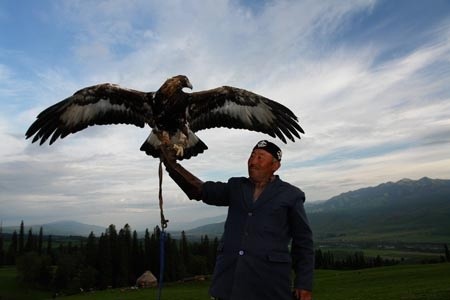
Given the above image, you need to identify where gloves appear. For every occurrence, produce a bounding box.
[158,144,203,200]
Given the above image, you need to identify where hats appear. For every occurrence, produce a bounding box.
[253,140,282,161]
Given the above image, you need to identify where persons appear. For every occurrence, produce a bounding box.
[158,140,316,300]
